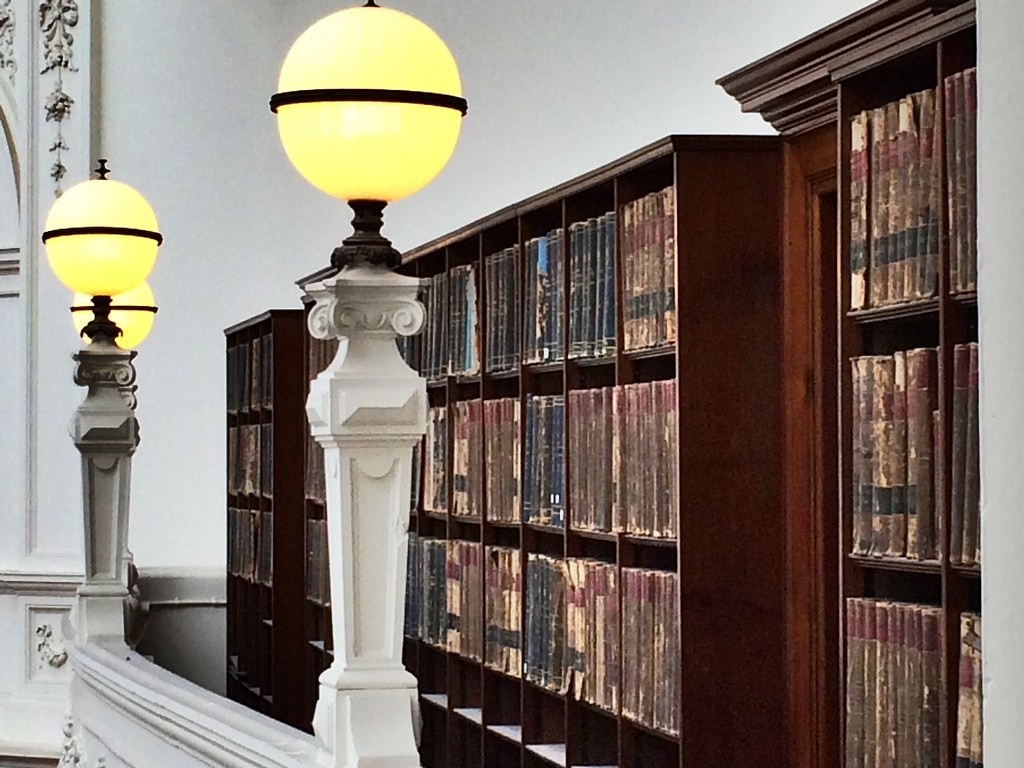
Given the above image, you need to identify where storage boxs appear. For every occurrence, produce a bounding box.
[223,0,982,768]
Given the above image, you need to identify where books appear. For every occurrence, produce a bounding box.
[305,336,339,606]
[403,186,680,740]
[227,335,273,588]
[847,65,983,768]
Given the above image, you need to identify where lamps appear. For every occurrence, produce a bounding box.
[270,1,469,268]
[41,159,163,351]
